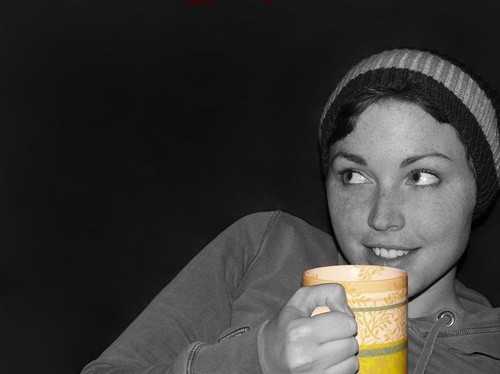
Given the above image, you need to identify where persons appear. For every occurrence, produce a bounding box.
[80,47,500,373]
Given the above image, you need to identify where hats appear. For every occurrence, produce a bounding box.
[317,47,499,220]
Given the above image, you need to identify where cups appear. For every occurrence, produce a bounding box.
[302,265,408,374]
